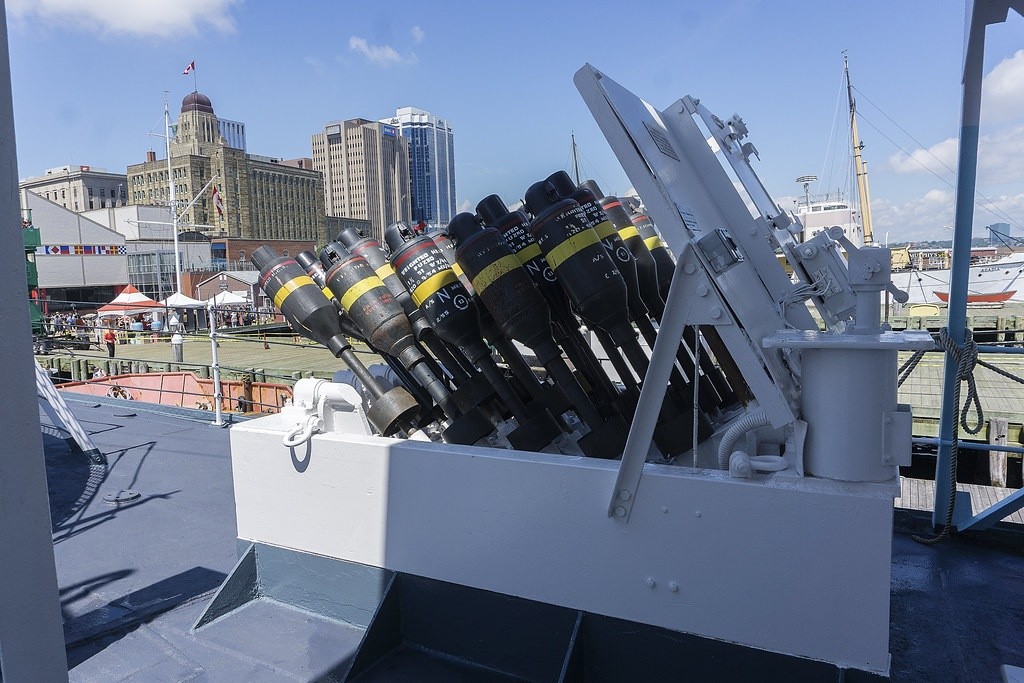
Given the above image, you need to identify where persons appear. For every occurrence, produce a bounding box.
[183,312,189,330]
[216,309,256,326]
[104,327,116,359]
[132,316,148,331]
[45,311,95,333]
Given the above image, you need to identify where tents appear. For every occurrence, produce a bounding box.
[204,289,254,318]
[97,283,165,334]
[156,291,208,329]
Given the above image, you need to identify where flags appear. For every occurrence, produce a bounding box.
[213,186,224,217]
